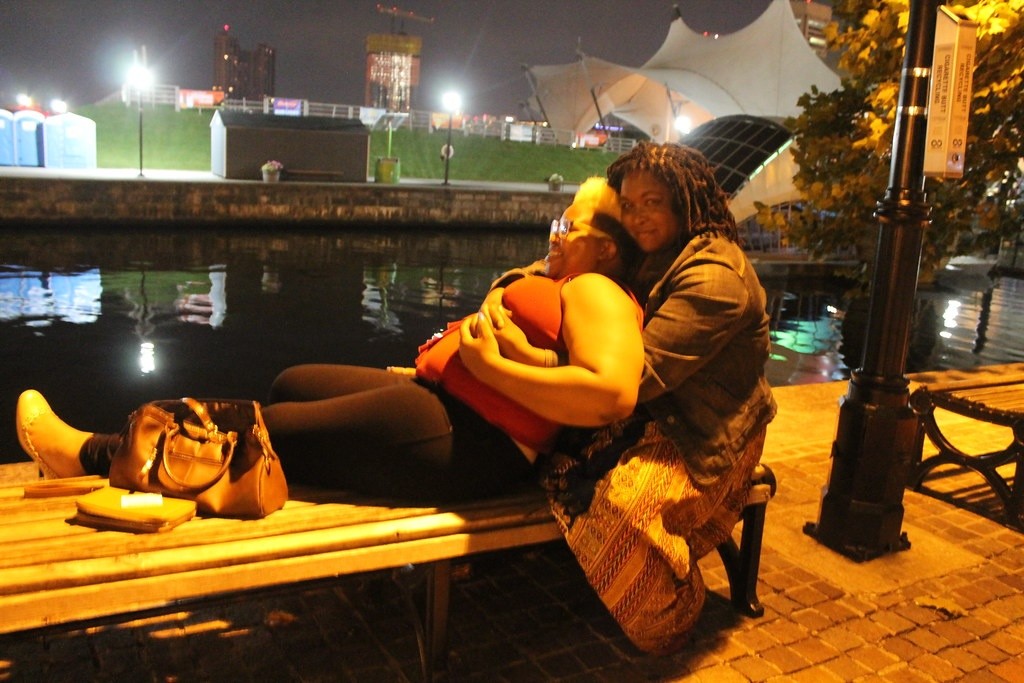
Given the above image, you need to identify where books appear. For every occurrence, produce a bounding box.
[24,475,196,535]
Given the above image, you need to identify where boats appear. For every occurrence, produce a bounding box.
[936,251,1000,291]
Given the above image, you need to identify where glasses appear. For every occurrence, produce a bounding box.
[550,219,600,240]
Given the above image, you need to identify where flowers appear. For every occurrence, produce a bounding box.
[262,160,283,174]
[549,173,563,183]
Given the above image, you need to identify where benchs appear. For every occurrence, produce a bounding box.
[912,375,1024,513]
[1,459,778,683]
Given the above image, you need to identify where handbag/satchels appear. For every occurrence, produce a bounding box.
[108,396,288,518]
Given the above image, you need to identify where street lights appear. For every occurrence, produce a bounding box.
[133,68,152,179]
[441,90,461,186]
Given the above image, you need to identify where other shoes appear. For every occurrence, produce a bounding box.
[17,390,66,481]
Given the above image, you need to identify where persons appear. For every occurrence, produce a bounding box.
[976,196,1019,232]
[459,143,777,653]
[15,176,644,507]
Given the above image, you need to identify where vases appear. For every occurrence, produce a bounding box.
[263,171,280,182]
[548,181,560,191]
